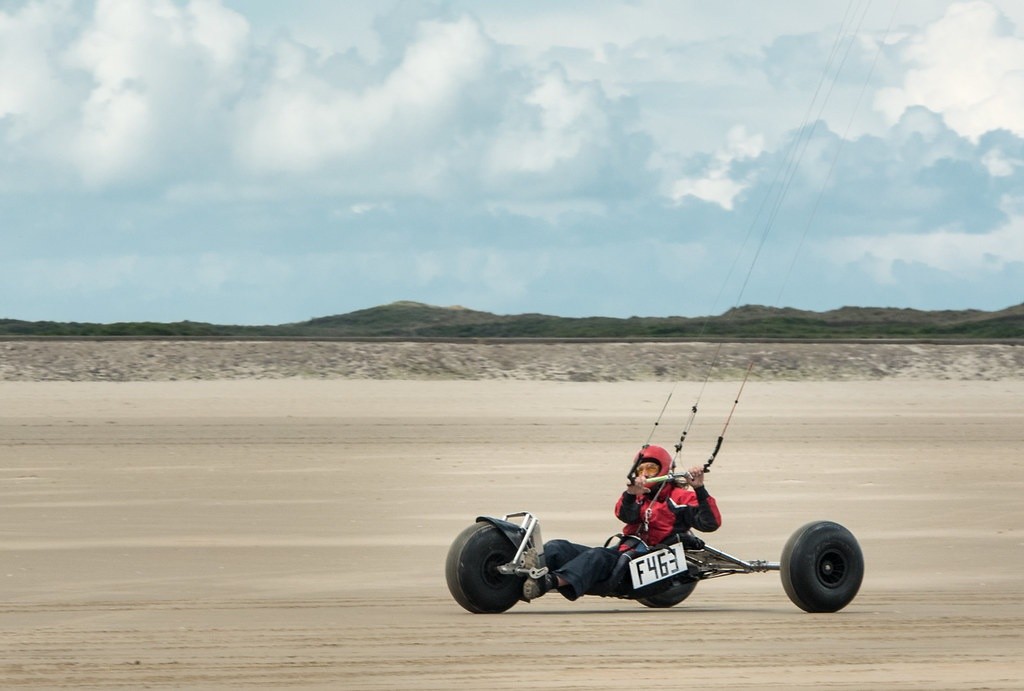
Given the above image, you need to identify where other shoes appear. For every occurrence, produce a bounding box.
[523,548,545,599]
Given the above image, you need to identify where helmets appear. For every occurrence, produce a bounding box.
[634,446,673,488]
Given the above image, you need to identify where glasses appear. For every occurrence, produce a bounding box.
[637,464,658,475]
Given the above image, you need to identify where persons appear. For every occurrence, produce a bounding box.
[522,445,721,601]
